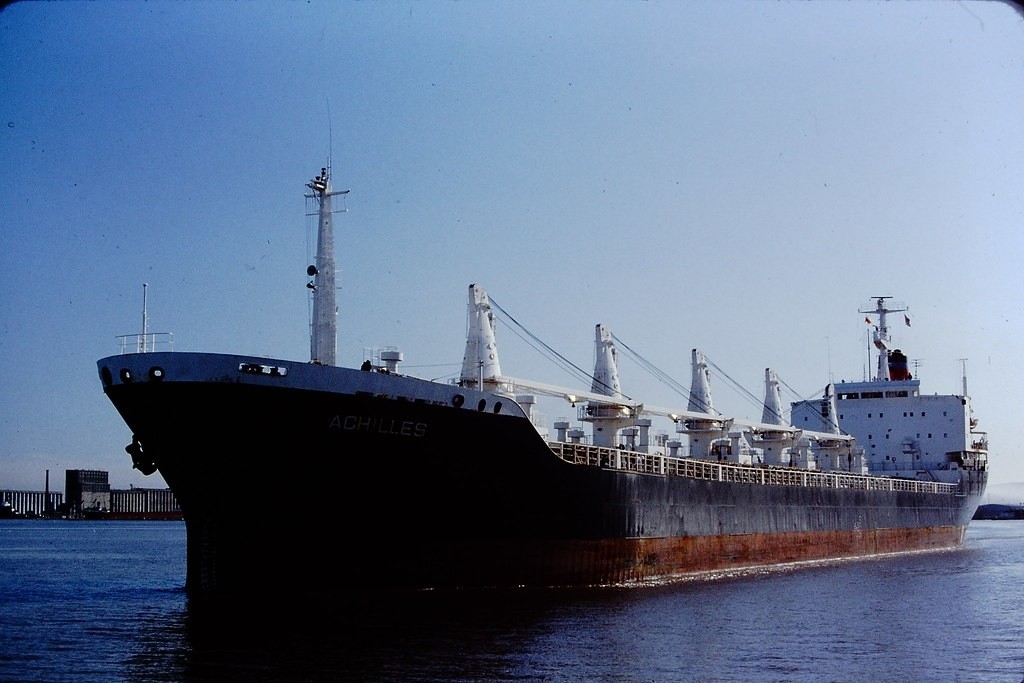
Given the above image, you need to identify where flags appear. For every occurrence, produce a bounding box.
[865,318,871,324]
[905,315,911,327]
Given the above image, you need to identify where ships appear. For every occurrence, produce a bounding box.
[96,99,987,609]
